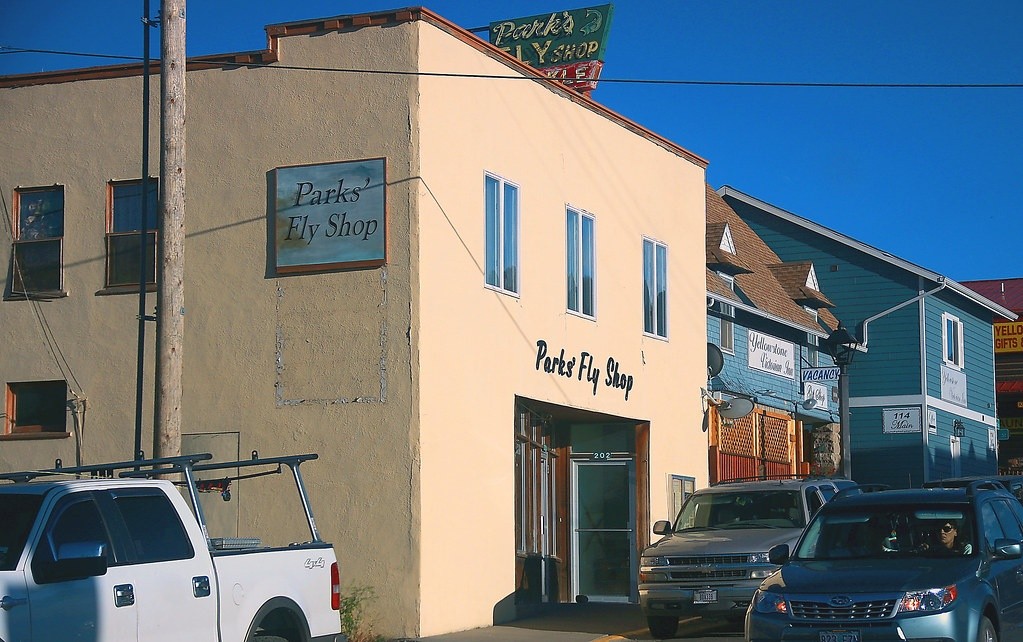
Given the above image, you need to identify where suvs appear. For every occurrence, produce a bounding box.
[639,474,865,639]
[744,473,1023,642]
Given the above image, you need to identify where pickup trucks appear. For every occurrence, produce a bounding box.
[0,450,344,642]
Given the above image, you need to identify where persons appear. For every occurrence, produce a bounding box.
[922,519,971,554]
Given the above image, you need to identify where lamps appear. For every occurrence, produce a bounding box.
[700,387,755,419]
[798,319,859,395]
[954,419,965,437]
[793,398,817,410]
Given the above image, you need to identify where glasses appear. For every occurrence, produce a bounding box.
[937,525,955,532]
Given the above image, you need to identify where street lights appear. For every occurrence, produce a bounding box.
[824,319,859,482]
